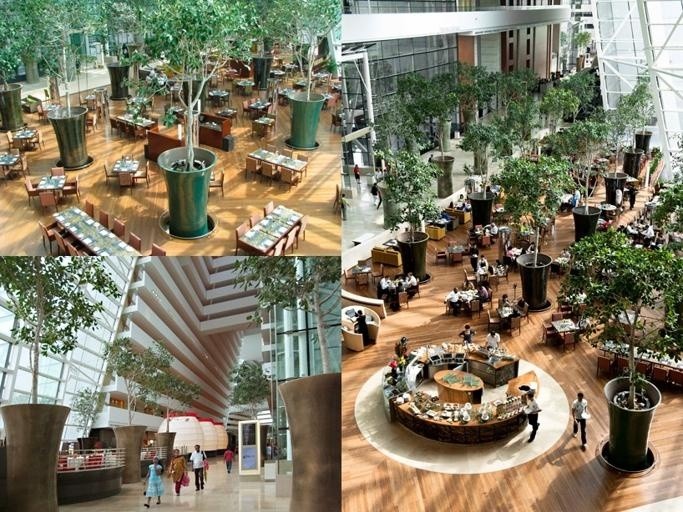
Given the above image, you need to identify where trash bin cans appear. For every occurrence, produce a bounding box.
[223,135,234,151]
[263,460,278,480]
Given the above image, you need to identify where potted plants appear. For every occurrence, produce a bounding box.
[100,339,201,484]
[364,53,657,311]
[556,227,683,479]
[1,258,124,510]
[1,1,343,240]
[228,358,273,466]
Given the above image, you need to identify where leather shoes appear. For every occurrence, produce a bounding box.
[196,487,204,491]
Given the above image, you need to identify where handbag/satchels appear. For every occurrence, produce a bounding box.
[180,474,190,486]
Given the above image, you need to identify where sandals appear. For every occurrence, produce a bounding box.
[144,502,160,508]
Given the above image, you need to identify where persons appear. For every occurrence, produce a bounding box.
[355,310,370,346]
[543,184,582,214]
[375,271,419,311]
[430,183,538,330]
[570,392,590,451]
[639,225,655,243]
[457,324,476,343]
[650,242,660,251]
[341,193,351,221]
[655,328,678,357]
[222,446,235,472]
[523,390,542,442]
[189,444,208,490]
[144,67,182,97]
[265,441,287,461]
[483,330,501,349]
[625,222,639,241]
[560,248,571,265]
[370,183,379,206]
[574,314,592,343]
[352,164,360,184]
[142,455,164,508]
[166,448,188,496]
[627,185,637,211]
[428,154,437,178]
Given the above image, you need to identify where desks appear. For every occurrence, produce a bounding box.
[380,343,538,443]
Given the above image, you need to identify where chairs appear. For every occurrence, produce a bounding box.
[237,204,305,256]
[54,229,90,257]
[595,322,683,384]
[541,311,585,352]
